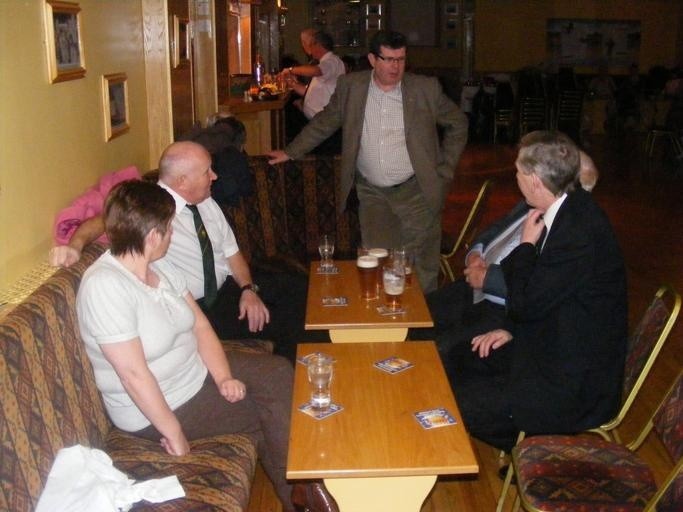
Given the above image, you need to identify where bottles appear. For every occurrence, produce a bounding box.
[253,54,264,86]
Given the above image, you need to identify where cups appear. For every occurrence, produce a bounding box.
[306,353,334,412]
[356,244,415,314]
[317,233,334,272]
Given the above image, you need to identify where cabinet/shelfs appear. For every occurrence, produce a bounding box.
[188,0,297,157]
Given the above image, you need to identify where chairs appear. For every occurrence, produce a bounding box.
[458,66,683,158]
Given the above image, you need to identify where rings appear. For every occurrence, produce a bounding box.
[240,390,244,393]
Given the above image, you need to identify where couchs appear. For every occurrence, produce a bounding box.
[0,116,318,512]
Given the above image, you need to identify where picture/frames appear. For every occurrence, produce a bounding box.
[43,0,87,84]
[101,72,132,144]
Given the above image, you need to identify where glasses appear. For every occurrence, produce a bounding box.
[376,55,406,64]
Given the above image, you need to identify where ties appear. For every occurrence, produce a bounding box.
[536,215,546,255]
[472,218,528,304]
[185,204,218,311]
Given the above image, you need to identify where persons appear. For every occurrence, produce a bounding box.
[263,27,471,296]
[75,175,340,512]
[46,137,331,366]
[276,31,346,153]
[592,59,683,132]
[408,148,599,357]
[445,126,629,485]
[288,28,321,119]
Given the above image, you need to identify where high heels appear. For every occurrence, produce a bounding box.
[290,477,338,511]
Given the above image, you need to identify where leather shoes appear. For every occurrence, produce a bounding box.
[497,463,517,485]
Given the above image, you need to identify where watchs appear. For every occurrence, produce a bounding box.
[289,66,293,73]
[241,282,261,294]
